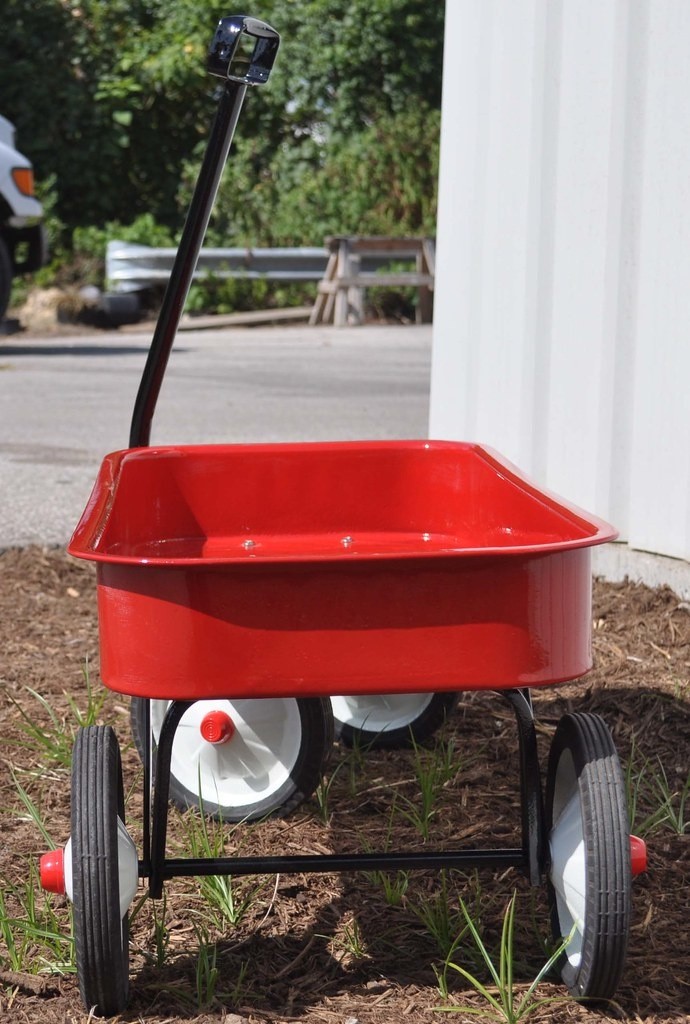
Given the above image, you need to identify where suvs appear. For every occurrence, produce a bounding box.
[0,114,46,319]
